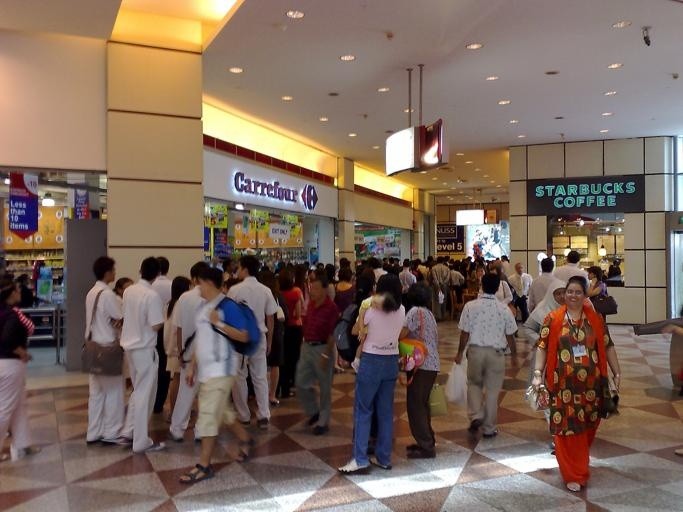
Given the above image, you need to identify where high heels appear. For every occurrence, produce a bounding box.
[270,400,281,408]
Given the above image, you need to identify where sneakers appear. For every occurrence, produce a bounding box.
[147,441,165,451]
[468,420,480,432]
[407,444,420,450]
[407,450,435,458]
[352,357,360,375]
[257,418,268,428]
[313,425,329,435]
[369,454,392,469]
[482,432,496,438]
[309,414,318,424]
[338,459,369,475]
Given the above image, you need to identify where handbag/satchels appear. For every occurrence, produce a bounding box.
[603,376,619,419]
[81,341,124,374]
[429,383,447,416]
[509,303,516,316]
[591,296,617,314]
[277,305,285,322]
[525,384,551,410]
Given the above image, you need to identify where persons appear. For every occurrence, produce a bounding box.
[660,307,683,456]
[0,272,38,460]
[86,247,622,492]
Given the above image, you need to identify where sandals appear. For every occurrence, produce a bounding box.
[566,482,580,491]
[180,464,214,483]
[236,439,255,462]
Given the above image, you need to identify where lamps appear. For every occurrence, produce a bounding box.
[42,192,55,207]
[564,236,571,256]
[598,229,606,256]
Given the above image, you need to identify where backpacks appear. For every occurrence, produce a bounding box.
[211,297,260,355]
[399,307,427,385]
[334,303,361,366]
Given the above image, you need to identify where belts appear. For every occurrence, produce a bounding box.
[308,341,326,345]
